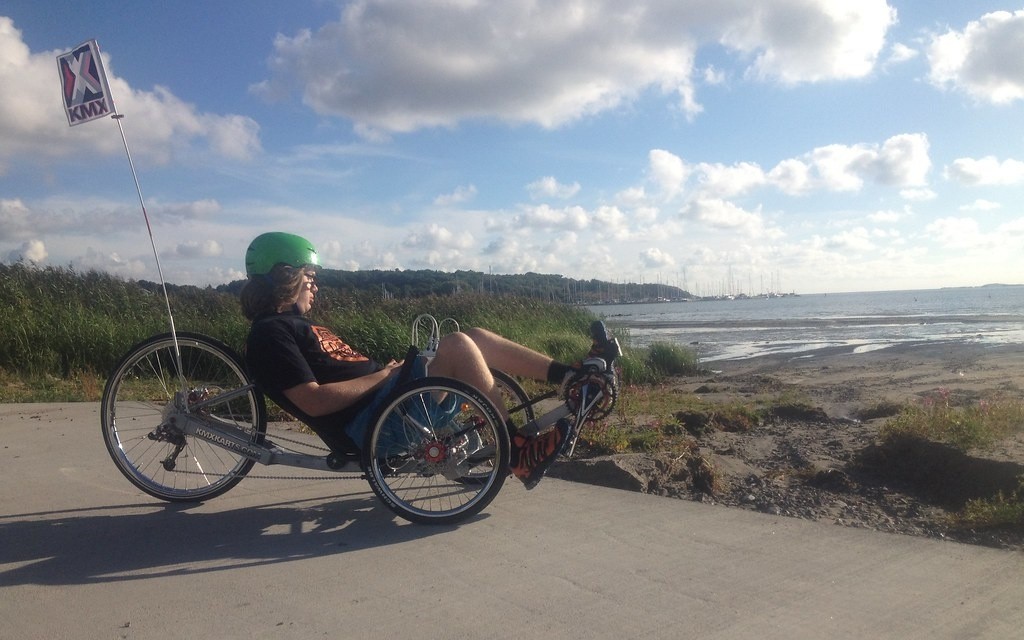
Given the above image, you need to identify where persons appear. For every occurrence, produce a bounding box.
[240,232,606,490]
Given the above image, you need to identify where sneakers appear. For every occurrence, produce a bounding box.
[512,417,571,490]
[574,320,609,402]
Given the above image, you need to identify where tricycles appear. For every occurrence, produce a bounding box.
[100,313,622,527]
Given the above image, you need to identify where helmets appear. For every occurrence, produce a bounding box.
[245,231,322,278]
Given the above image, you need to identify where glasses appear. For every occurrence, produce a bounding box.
[301,271,317,286]
